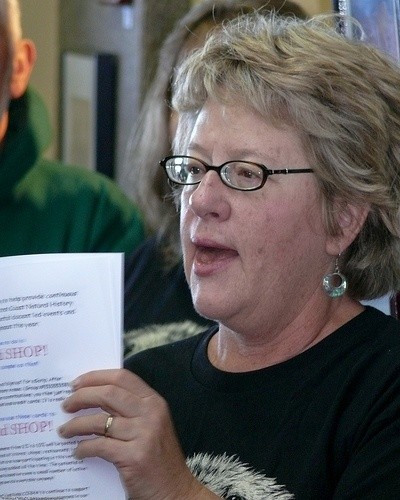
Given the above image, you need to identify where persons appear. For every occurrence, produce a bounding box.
[0,1,162,329]
[55,10,400,500]
[123,0,313,357]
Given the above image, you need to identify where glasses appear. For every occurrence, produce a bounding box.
[158,155,318,192]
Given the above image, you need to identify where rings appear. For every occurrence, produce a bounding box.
[104,416,114,435]
